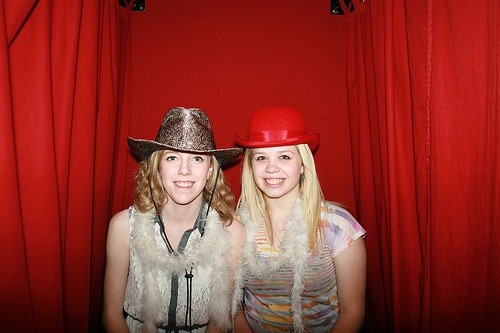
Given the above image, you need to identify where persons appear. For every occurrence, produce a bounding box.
[231,106,367,333]
[103,106,245,333]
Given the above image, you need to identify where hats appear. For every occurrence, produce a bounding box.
[234,104,321,154]
[127,105,244,166]
